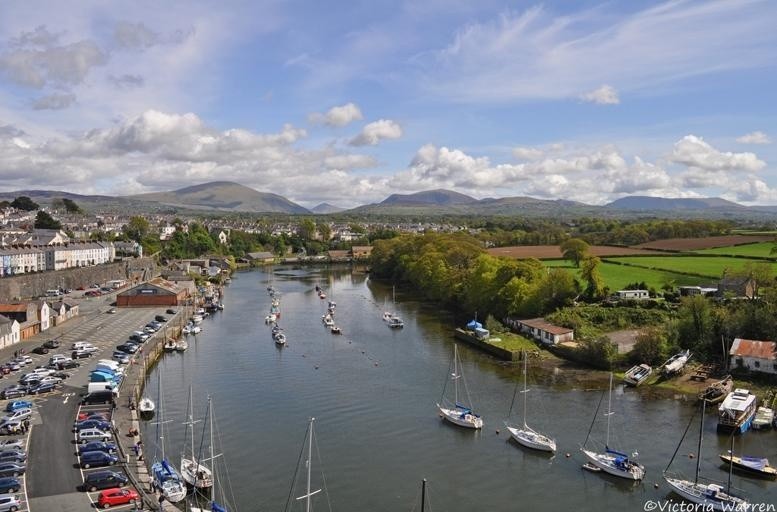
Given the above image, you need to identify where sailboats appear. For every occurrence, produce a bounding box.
[501,348,558,455]
[663,395,753,511]
[408,477,439,510]
[434,344,484,431]
[578,372,646,482]
[138,355,340,511]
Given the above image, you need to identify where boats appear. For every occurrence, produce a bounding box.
[719,447,776,478]
[163,277,231,354]
[690,361,719,380]
[697,373,776,437]
[382,310,403,329]
[264,284,287,347]
[315,285,340,334]
[623,364,652,386]
[656,347,694,378]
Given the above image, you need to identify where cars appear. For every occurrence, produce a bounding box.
[109,301,116,306]
[44,279,125,298]
[107,307,116,314]
[129,315,167,342]
[0,339,141,511]
[166,308,177,314]
